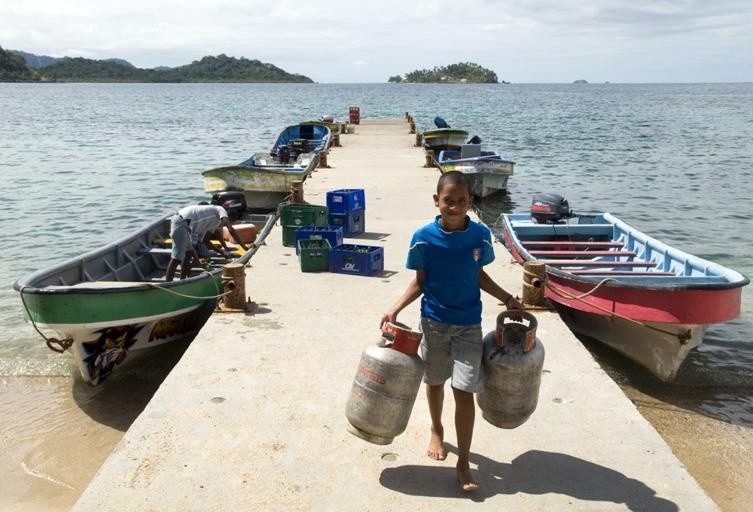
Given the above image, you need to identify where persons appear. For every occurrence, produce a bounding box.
[189,202,227,258]
[379,172,523,492]
[165,200,257,282]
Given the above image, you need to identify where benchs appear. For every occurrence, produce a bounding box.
[139,238,250,262]
[517,238,677,275]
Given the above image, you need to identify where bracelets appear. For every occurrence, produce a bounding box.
[504,295,512,305]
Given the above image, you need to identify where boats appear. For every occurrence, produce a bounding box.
[11,202,277,388]
[421,126,517,199]
[200,117,344,211]
[500,210,751,386]
